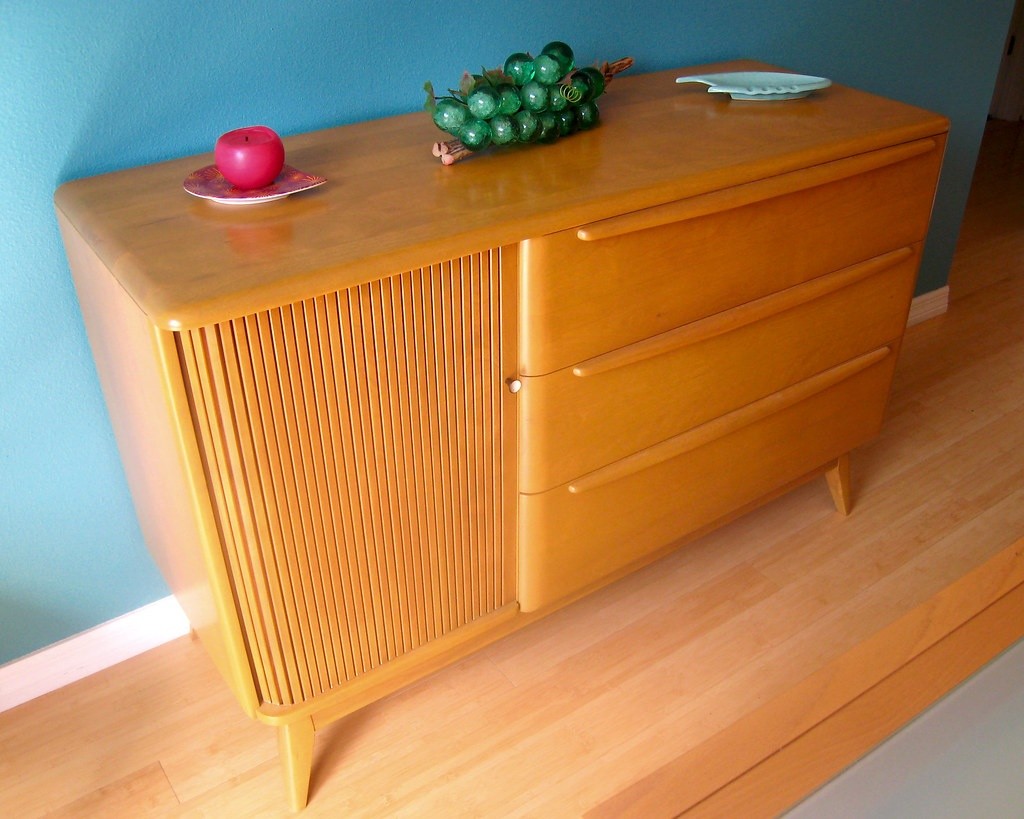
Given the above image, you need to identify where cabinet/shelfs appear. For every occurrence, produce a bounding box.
[51,61,948,816]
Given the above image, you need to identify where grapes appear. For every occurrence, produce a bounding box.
[432,41,606,150]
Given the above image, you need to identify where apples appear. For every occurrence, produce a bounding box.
[215,125,285,189]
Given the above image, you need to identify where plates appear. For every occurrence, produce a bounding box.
[676,71,831,101]
[183,162,327,204]
